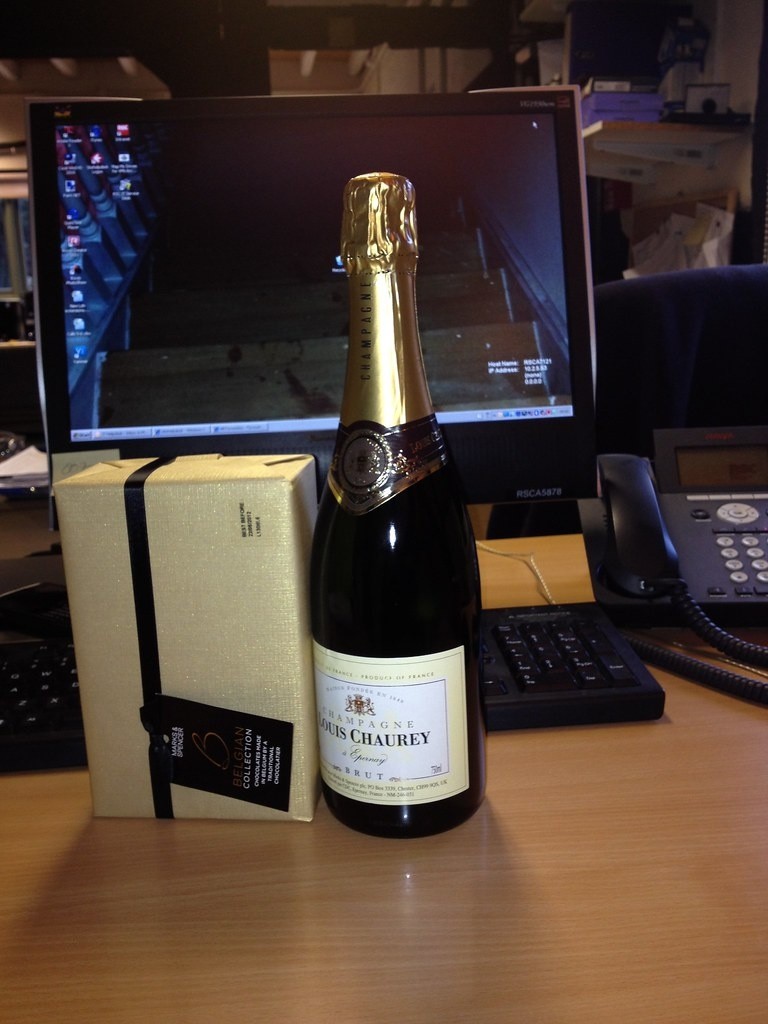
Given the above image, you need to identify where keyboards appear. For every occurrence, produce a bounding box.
[1,600,667,775]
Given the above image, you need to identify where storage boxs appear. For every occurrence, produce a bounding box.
[53,453,320,823]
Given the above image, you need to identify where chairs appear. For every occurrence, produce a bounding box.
[487,264,768,539]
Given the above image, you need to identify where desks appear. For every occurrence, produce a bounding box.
[0,502,768,1024]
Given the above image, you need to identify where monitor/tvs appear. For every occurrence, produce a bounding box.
[23,84,597,533]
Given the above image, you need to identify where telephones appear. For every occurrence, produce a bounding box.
[576,426,768,627]
